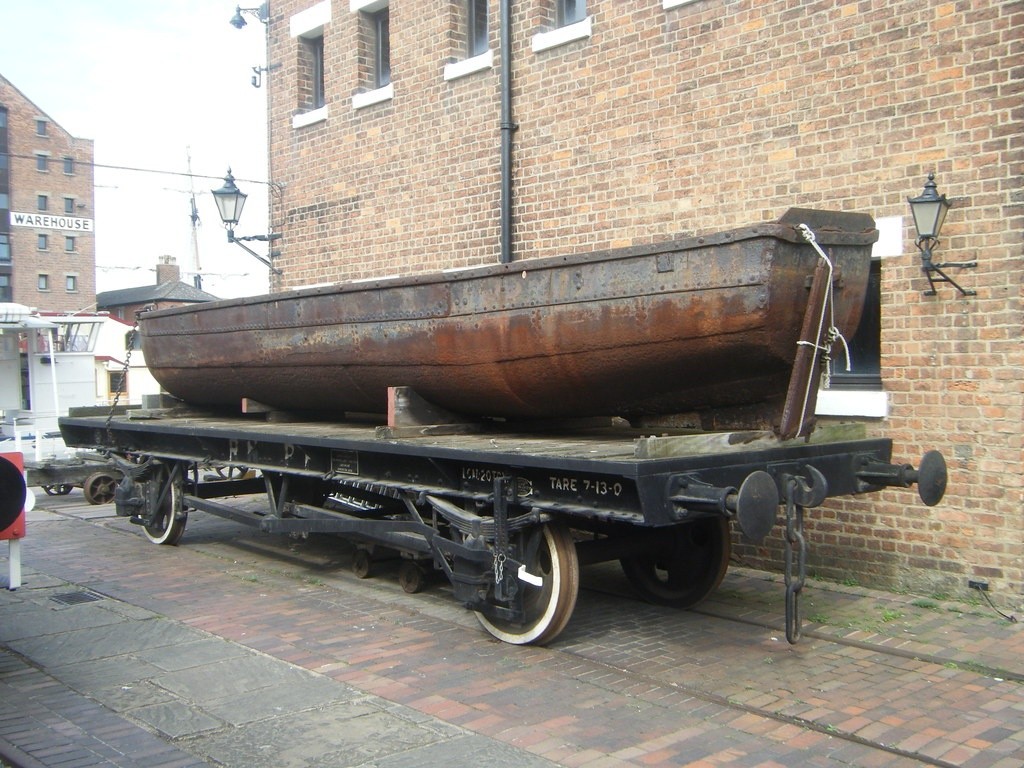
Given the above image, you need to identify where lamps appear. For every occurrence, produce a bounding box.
[211,168,283,274]
[907,172,977,296]
[252,63,281,88]
[229,3,267,29]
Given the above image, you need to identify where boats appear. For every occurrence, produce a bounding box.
[137,205,880,433]
